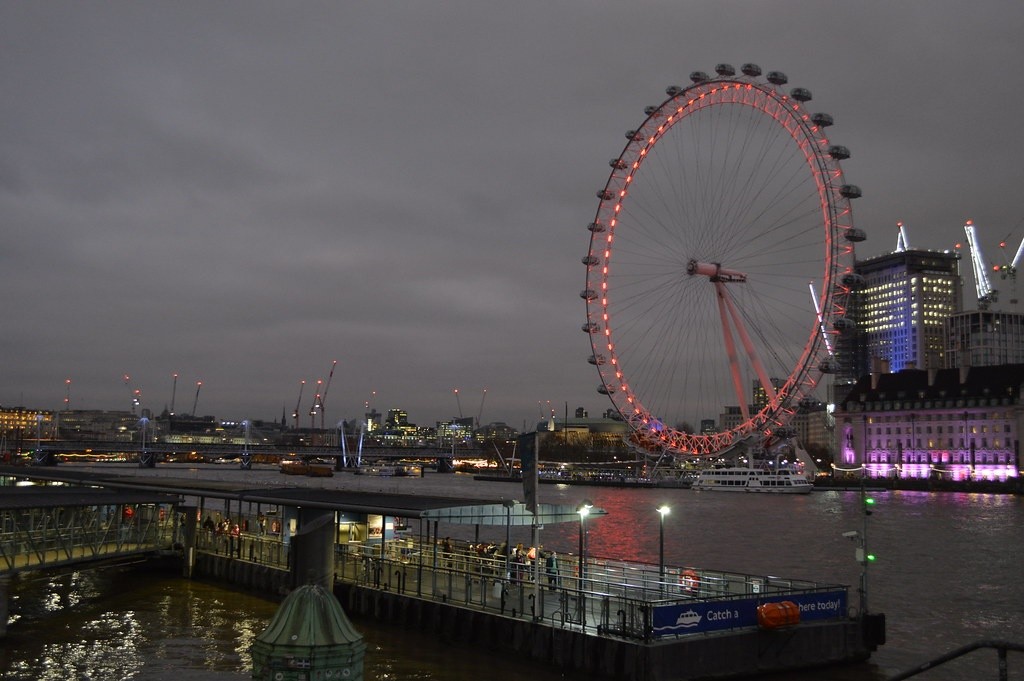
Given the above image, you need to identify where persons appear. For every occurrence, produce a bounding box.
[443,536,560,592]
[203,512,267,552]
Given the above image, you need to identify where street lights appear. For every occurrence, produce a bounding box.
[672,456,822,475]
[656,504,674,604]
[863,415,868,478]
[964,410,969,479]
[910,413,916,477]
[575,499,594,625]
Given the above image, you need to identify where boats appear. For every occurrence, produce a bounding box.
[278,460,337,479]
[473,467,523,482]
[520,466,651,485]
[213,457,228,464]
[691,468,814,495]
[352,464,423,477]
[620,468,701,489]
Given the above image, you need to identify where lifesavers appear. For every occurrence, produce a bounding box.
[679,571,698,592]
